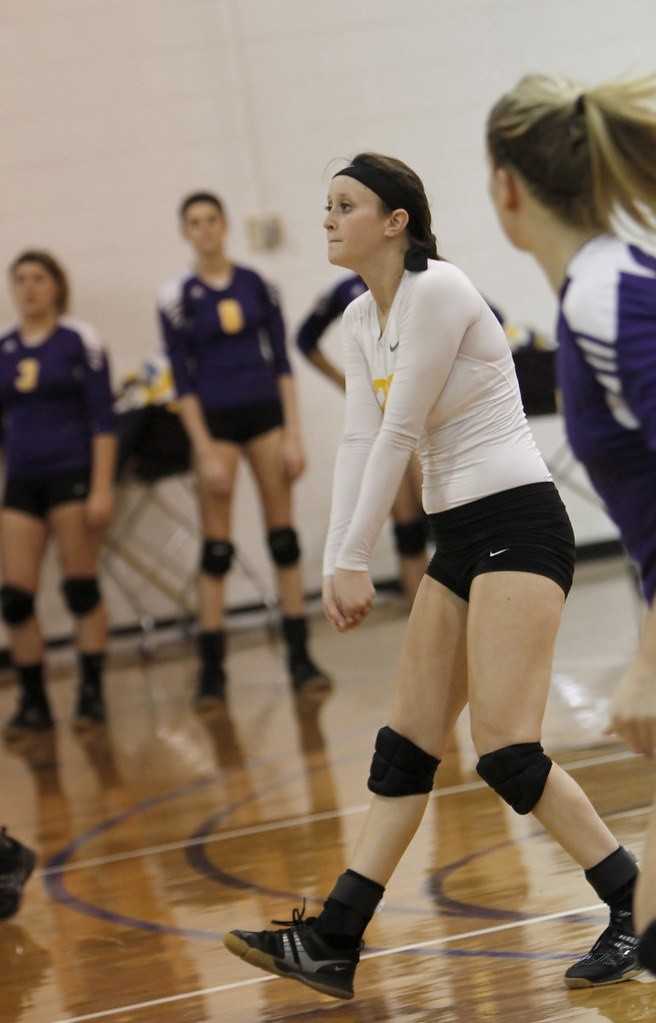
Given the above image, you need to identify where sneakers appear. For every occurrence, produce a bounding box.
[564,909,646,988]
[224,900,359,1000]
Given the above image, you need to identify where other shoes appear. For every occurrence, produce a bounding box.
[4,700,54,741]
[72,690,106,731]
[195,666,224,709]
[289,658,329,693]
[0,825,36,918]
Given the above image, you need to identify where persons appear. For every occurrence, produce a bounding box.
[485,73,656,971]
[0,826,37,920]
[158,193,330,709]
[0,253,119,738]
[295,271,506,616]
[224,153,644,999]
